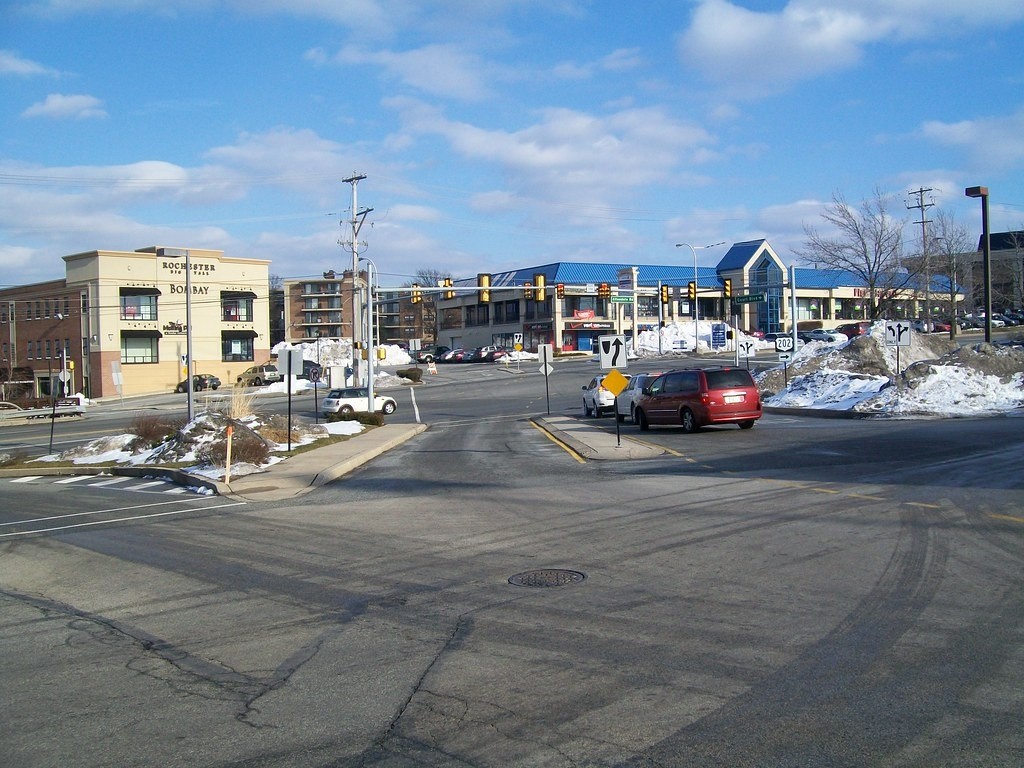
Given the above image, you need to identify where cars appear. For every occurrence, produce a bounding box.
[942,310,1024,329]
[932,320,952,332]
[390,341,503,362]
[279,360,323,379]
[731,325,765,341]
[177,374,221,393]
[757,331,792,350]
[911,319,935,334]
[486,347,514,362]
[813,328,849,344]
[789,331,827,345]
[614,371,668,425]
[835,322,871,341]
[580,373,633,417]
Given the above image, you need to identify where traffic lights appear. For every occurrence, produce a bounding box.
[661,319,667,328]
[377,348,386,361]
[599,288,605,300]
[354,341,361,351]
[602,284,608,289]
[557,283,564,299]
[660,284,668,304]
[723,279,732,298]
[524,283,533,299]
[687,282,695,301]
[604,288,610,299]
[727,331,735,339]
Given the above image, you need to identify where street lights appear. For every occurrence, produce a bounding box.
[156,248,197,422]
[965,186,993,343]
[357,256,381,348]
[676,243,700,353]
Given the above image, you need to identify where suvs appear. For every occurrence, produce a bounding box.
[636,366,763,432]
[321,386,398,415]
[236,364,280,386]
